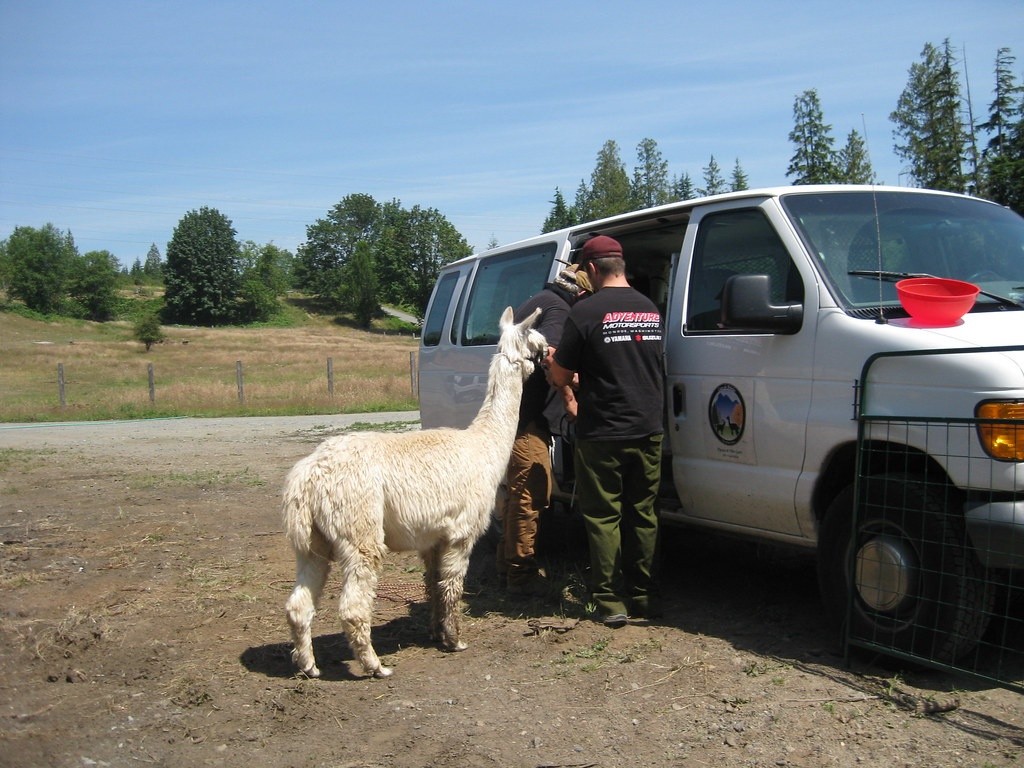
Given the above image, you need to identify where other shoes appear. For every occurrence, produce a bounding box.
[599,612,628,624]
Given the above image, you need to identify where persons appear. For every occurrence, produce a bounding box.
[493,264,596,600]
[547,235,668,624]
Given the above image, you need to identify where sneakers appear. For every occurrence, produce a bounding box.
[498,566,554,591]
[506,573,563,598]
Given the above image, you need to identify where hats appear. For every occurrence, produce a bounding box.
[574,236,622,274]
[558,264,594,294]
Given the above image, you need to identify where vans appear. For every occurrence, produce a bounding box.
[411,183,1023,668]
